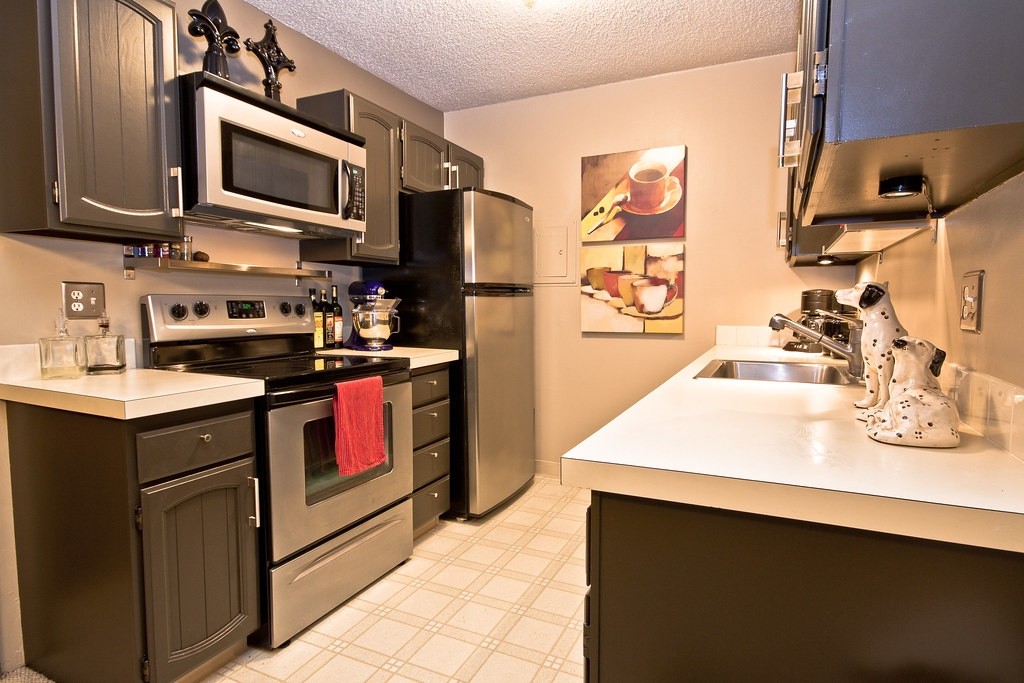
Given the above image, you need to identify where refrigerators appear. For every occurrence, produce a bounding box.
[363,187,535,523]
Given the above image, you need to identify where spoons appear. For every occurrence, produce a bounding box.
[587,194,628,234]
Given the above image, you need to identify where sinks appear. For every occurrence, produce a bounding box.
[693,359,867,387]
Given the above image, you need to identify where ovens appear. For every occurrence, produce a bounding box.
[177,71,367,240]
[247,371,414,652]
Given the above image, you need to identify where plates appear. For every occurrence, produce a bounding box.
[614,177,682,216]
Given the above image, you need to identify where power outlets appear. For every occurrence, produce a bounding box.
[960,269,985,334]
[62,281,105,320]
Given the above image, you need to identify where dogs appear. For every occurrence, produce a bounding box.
[857,335,968,448]
[835,279,910,408]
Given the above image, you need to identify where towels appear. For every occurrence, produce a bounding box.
[332,376,388,476]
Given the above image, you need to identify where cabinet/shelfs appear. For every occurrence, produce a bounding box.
[0,0,185,245]
[5,402,261,683]
[297,88,484,267]
[582,492,1023,683]
[778,0,1024,269]
[410,367,451,537]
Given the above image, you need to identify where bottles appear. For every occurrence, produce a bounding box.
[39,308,85,381]
[309,285,343,351]
[123,236,192,261]
[84,309,126,376]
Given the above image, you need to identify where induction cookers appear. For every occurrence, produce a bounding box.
[138,293,410,392]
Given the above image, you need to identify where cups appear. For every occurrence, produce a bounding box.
[629,161,679,210]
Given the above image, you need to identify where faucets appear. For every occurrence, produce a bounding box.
[768,308,866,380]
